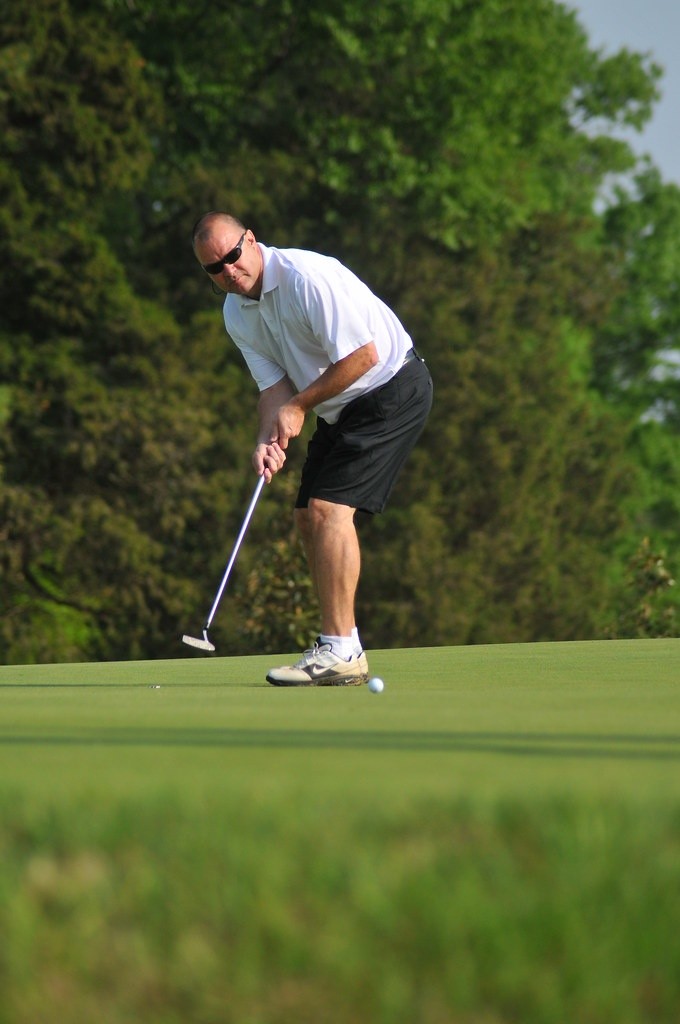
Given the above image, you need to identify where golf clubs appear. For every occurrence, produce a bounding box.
[178,471,265,652]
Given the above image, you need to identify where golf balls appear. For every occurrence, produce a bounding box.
[368,677,384,694]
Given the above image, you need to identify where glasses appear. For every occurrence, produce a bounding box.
[202,232,247,276]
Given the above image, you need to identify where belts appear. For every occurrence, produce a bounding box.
[402,349,416,368]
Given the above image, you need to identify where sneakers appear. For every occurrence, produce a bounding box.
[265,636,370,687]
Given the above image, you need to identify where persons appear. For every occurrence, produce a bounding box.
[191,211,431,686]
